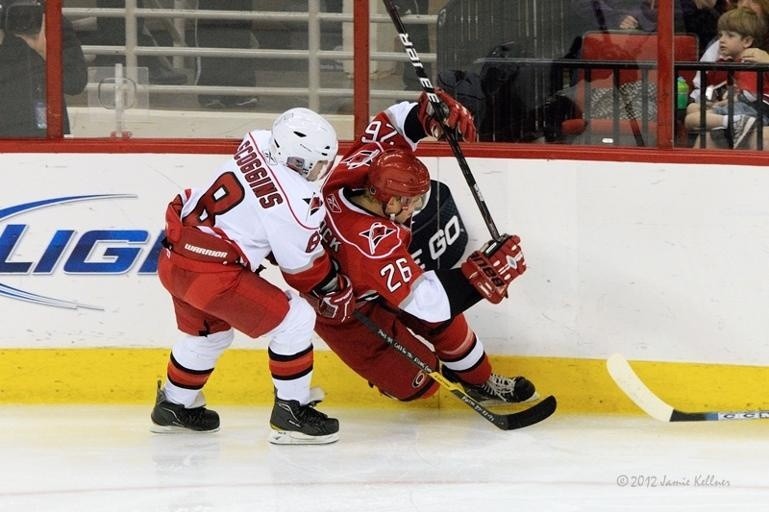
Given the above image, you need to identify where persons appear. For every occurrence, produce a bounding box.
[149,107,355,437]
[308,86,536,403]
[1,2,768,154]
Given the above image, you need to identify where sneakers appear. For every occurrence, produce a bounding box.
[733,114,757,149]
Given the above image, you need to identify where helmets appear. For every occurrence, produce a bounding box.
[367,149,431,202]
[269,107,338,183]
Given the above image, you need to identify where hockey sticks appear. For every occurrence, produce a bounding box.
[606,354,769,423]
[354,310,556,430]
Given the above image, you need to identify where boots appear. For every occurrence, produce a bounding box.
[270,384,339,435]
[150,381,219,431]
[442,363,535,403]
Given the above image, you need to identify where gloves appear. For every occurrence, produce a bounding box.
[461,233,527,304]
[418,86,476,144]
[315,272,356,323]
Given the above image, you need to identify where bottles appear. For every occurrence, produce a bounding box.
[677,76,690,109]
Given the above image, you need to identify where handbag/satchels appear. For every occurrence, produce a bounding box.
[582,79,657,120]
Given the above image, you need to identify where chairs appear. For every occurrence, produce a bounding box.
[560,30,700,139]
